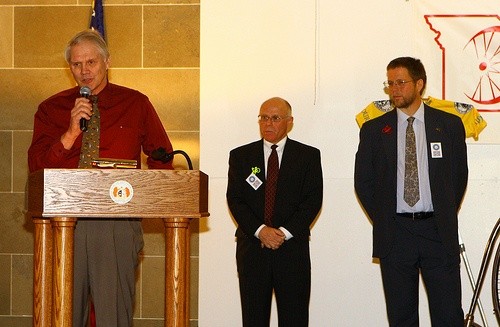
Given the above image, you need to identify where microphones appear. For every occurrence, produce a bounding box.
[80,86,91,132]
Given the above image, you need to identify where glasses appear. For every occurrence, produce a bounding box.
[382,80,413,88]
[258,115,286,123]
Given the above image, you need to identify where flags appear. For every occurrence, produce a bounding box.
[90,0,104,36]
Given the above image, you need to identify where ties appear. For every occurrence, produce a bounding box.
[403,117,420,207]
[78,95,100,168]
[265,144,279,228]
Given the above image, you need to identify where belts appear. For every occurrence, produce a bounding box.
[396,211,435,221]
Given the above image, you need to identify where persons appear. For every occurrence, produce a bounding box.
[27,29,174,327]
[226,96,323,327]
[353,56,468,327]
